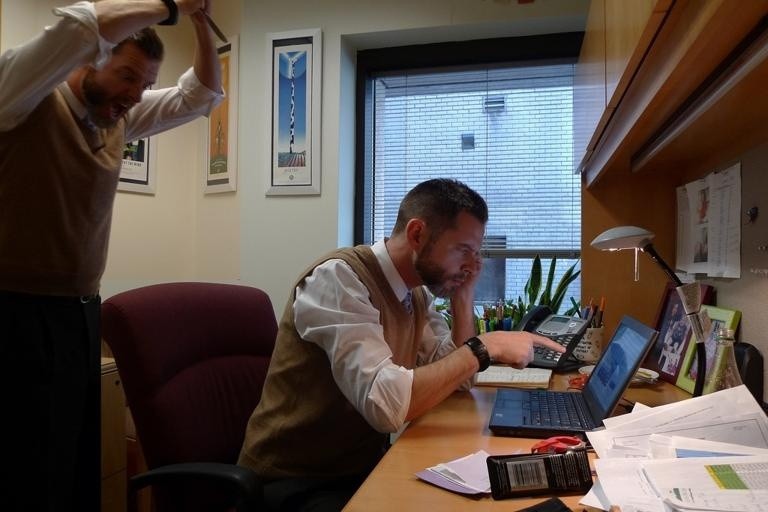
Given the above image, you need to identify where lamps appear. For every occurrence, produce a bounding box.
[590,225,708,399]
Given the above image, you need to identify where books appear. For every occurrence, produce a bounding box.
[584,383,768,512]
[472,366,552,390]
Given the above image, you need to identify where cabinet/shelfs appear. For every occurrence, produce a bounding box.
[570,0,768,191]
[97,354,128,511]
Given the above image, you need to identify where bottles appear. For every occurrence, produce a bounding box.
[705,328,743,395]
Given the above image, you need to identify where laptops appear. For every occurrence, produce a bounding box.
[489,314,660,439]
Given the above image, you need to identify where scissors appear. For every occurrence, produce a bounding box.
[529,436,594,453]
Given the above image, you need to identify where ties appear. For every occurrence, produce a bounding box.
[401,293,414,315]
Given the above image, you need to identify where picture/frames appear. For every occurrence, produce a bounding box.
[203,32,238,197]
[262,27,323,197]
[116,73,158,196]
[640,279,742,396]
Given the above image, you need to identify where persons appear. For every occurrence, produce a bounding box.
[234,178,569,512]
[0,0,227,512]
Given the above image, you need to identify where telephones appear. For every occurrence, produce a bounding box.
[510,303,589,368]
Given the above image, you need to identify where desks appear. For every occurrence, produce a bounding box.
[340,353,768,512]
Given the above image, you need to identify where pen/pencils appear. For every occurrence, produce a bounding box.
[479,297,517,333]
[570,296,605,328]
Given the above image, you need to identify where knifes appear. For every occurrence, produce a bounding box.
[199,8,229,44]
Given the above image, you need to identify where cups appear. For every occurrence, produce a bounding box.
[571,327,605,363]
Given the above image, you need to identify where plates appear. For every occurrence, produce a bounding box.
[578,365,660,386]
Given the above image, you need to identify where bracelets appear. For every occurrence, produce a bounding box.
[463,337,490,373]
[156,0,178,25]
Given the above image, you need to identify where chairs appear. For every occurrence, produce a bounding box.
[100,281,279,512]
